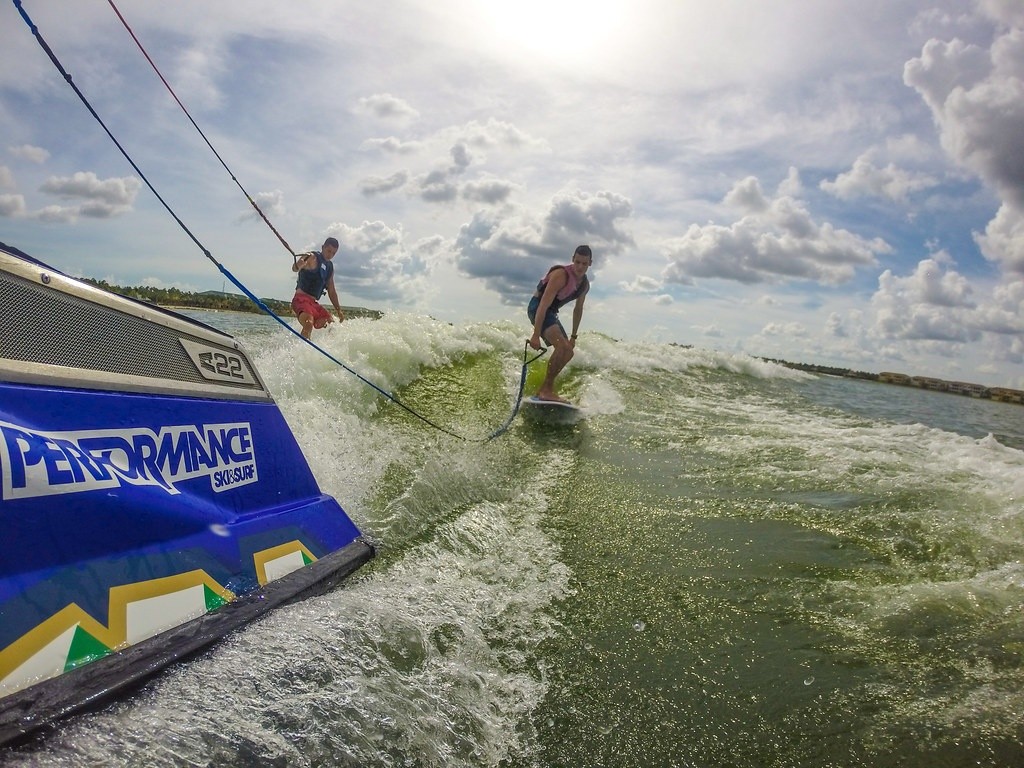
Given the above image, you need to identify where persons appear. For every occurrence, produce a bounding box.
[292,238,344,339]
[527,245,592,403]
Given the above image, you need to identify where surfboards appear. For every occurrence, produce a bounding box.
[526,396,581,411]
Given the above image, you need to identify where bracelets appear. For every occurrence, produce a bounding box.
[571,335,577,339]
